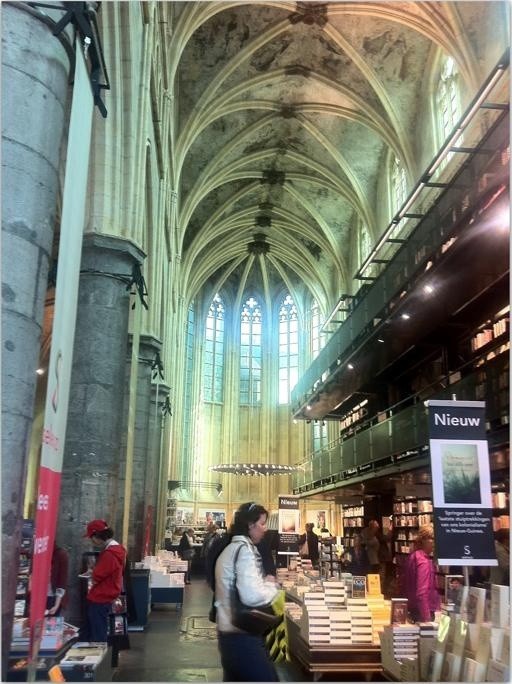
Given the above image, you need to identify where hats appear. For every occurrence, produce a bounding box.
[83,519,109,538]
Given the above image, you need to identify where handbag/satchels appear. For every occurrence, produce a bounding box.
[299,540,309,557]
[230,586,278,637]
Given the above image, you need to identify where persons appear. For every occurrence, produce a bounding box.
[84,517,126,643]
[180,503,281,681]
[22,541,69,618]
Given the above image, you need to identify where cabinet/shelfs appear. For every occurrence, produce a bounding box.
[343,498,436,574]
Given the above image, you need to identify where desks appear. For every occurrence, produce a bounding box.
[286,614,384,682]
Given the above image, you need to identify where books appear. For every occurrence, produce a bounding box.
[278,491,511,682]
[133,549,188,588]
[9,561,127,681]
